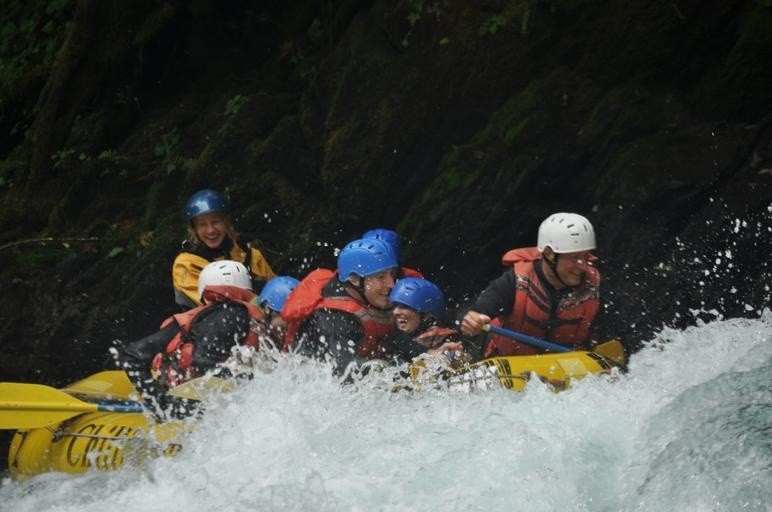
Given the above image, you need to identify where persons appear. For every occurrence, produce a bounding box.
[453,211,602,361]
[278,236,465,392]
[171,188,276,310]
[389,275,464,369]
[121,258,260,425]
[188,275,300,385]
[363,229,426,279]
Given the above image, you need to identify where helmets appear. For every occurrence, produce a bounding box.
[537,211,596,254]
[198,260,299,313]
[338,228,444,320]
[186,188,224,220]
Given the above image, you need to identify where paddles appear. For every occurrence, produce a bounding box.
[0,380,146,431]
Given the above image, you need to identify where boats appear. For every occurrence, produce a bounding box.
[8,350,629,481]
